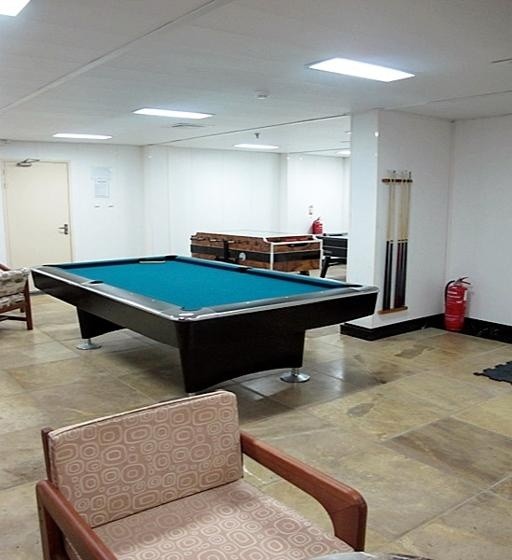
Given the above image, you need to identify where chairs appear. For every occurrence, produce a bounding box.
[37,389,372,560]
[1,264,33,331]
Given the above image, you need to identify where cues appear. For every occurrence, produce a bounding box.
[382,168,413,311]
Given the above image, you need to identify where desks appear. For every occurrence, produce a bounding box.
[30,253,380,399]
[185,232,347,279]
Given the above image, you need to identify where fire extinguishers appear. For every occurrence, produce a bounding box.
[312,216,323,235]
[443,277,471,332]
